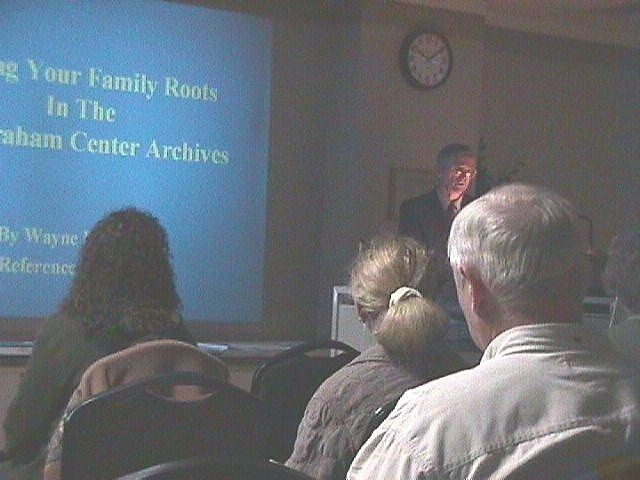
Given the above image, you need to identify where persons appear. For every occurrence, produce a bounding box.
[0,206,202,480]
[603,223,640,370]
[345,181,640,480]
[396,141,483,314]
[267,234,484,480]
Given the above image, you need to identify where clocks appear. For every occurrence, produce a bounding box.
[399,30,452,91]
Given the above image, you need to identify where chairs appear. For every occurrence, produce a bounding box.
[248,340,363,461]
[102,460,317,480]
[60,372,280,476]
